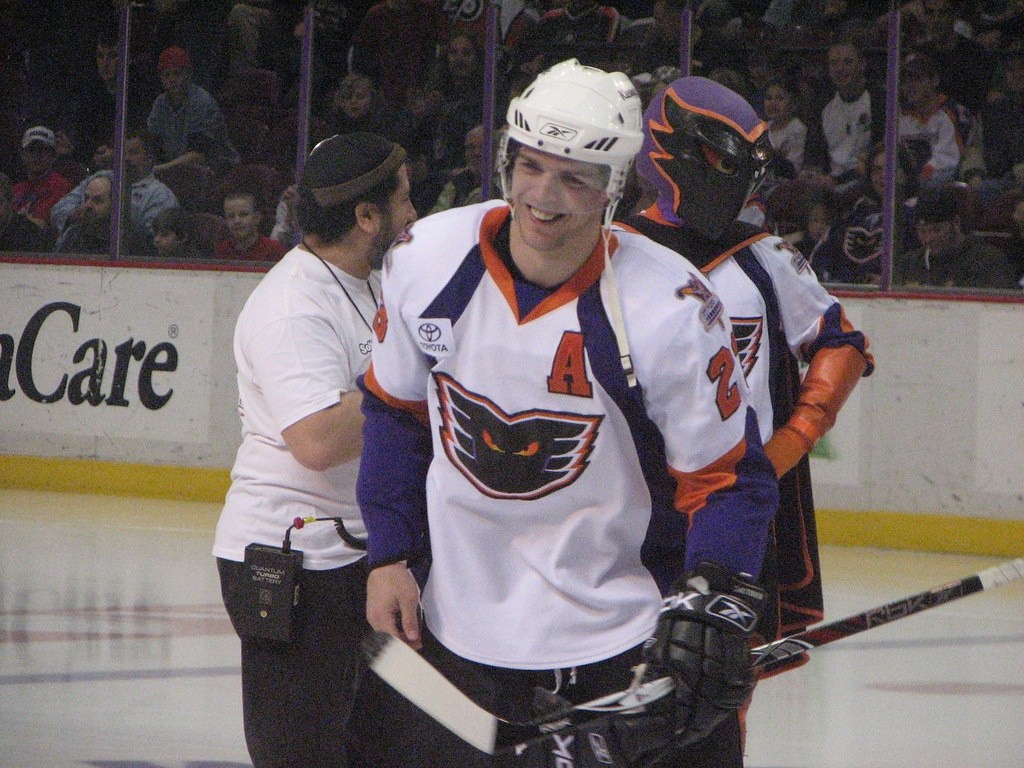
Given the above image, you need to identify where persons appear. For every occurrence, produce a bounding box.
[0,1,1024,289]
[356,57,876,768]
[213,131,416,768]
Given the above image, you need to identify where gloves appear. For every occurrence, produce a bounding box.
[762,343,869,481]
[603,558,771,768]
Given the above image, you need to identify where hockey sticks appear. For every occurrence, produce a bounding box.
[356,555,1024,757]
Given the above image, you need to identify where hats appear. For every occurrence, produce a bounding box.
[21,126,56,149]
[304,131,408,208]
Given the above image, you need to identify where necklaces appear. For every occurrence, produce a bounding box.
[302,240,378,333]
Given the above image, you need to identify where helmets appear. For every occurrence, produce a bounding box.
[634,75,776,238]
[496,57,646,200]
